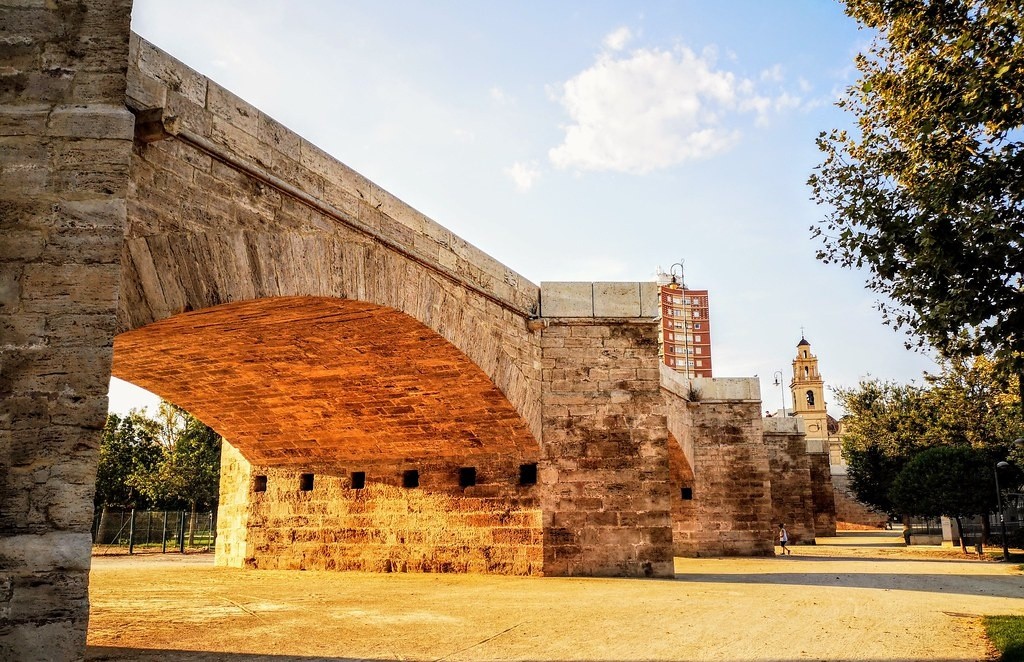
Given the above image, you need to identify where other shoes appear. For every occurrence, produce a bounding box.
[781,553,785,555]
[788,550,791,554]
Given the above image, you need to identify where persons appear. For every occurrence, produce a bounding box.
[776,524,790,555]
[766,411,772,417]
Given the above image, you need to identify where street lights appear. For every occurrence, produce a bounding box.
[773,371,787,418]
[993,461,1009,562]
[670,258,690,378]
